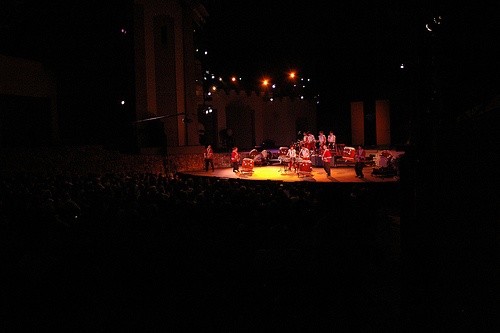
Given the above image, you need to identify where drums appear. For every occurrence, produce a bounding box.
[342,146,356,161]
[279,158,290,166]
[278,147,289,156]
[241,158,254,171]
[299,161,313,174]
[293,160,304,170]
[328,142,335,151]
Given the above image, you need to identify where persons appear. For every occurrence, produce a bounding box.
[293,129,336,155]
[299,146,310,160]
[286,145,297,171]
[353,145,366,179]
[321,145,332,178]
[203,145,215,174]
[0,145,375,333]
[230,146,240,173]
